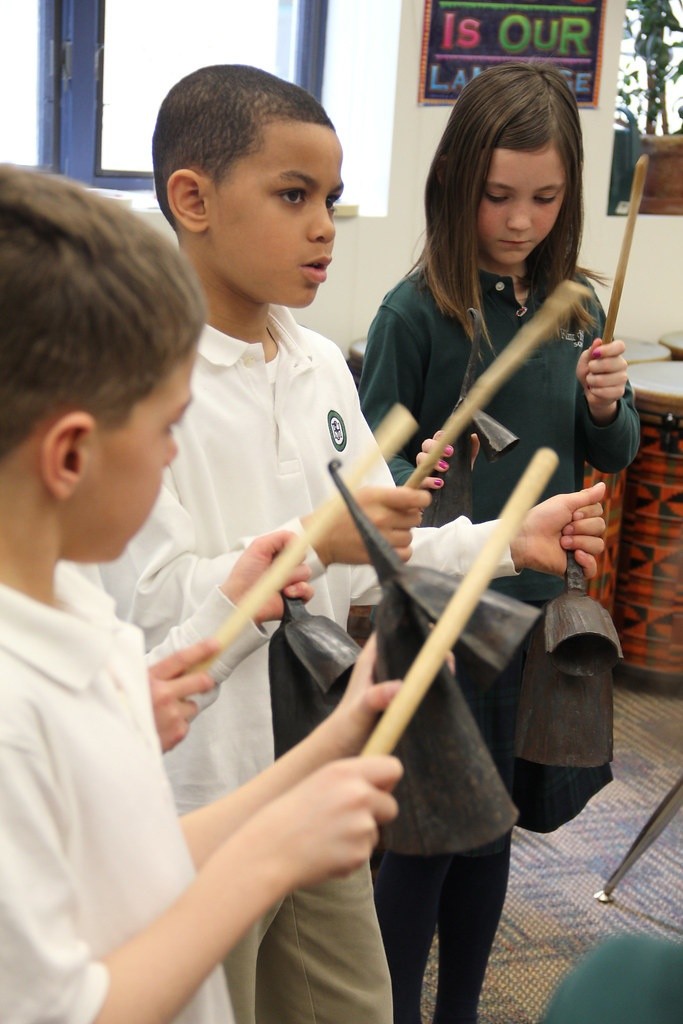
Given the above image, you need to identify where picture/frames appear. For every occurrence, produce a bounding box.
[416,0,607,108]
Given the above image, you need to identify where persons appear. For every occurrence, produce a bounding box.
[97,64,606,1024]
[0,164,454,1024]
[360,66,640,1024]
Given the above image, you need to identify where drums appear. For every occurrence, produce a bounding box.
[583,329,683,683]
